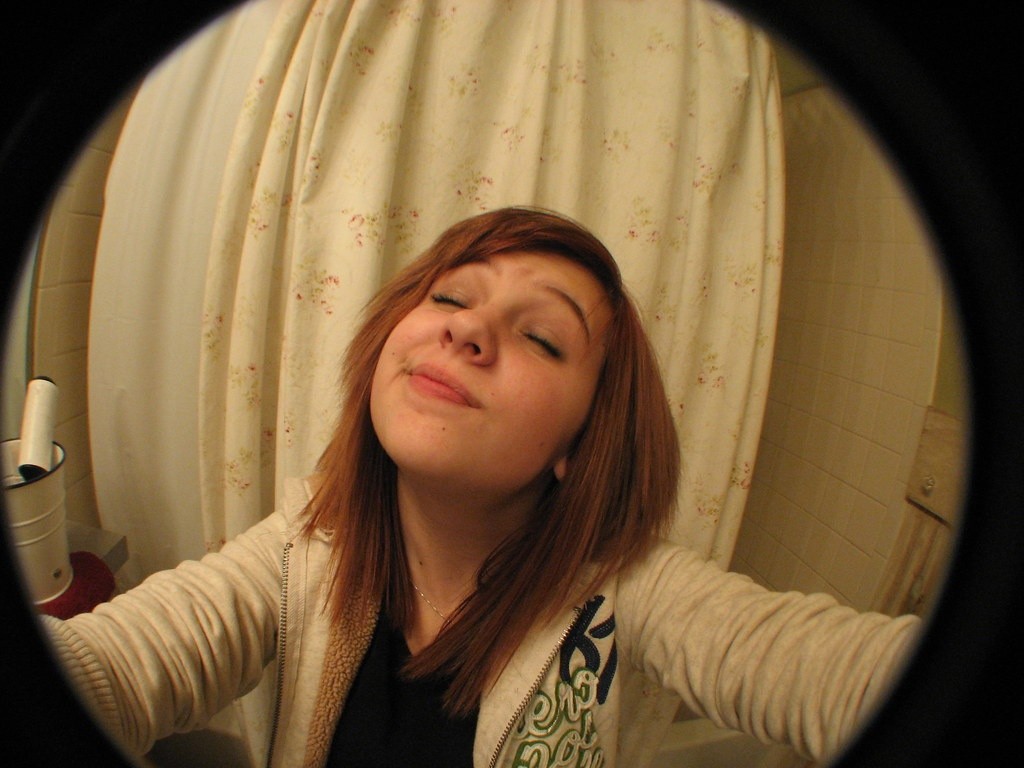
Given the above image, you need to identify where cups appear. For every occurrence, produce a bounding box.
[0,437,73,605]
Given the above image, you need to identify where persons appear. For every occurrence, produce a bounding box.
[44,203,924,768]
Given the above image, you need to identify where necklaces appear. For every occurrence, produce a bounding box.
[406,576,453,627]
